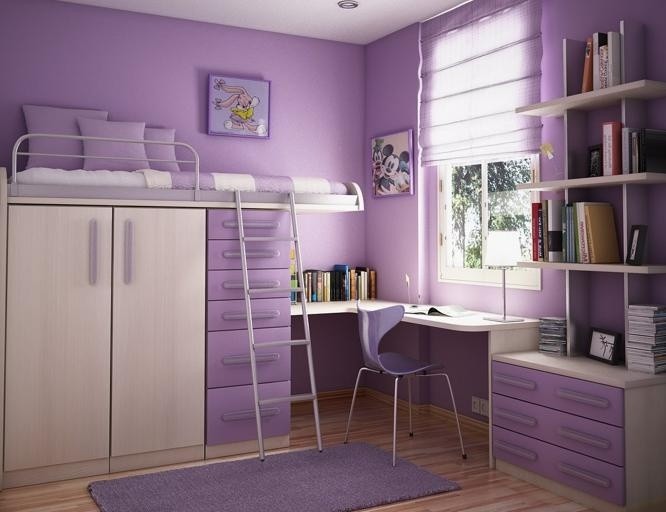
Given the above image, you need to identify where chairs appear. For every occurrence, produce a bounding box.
[343,296,468,468]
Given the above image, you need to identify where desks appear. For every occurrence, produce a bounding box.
[290,299,544,472]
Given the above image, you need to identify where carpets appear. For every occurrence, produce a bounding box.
[87,444,461,511]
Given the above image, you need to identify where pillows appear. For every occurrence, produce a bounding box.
[21,100,108,170]
[144,126,180,172]
[75,116,152,172]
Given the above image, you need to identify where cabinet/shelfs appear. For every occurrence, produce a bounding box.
[491,350,666,512]
[0,198,205,493]
[505,37,666,372]
[204,202,294,458]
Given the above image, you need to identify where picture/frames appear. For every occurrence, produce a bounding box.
[585,143,604,179]
[625,223,648,265]
[368,128,413,200]
[206,71,272,139]
[585,325,622,366]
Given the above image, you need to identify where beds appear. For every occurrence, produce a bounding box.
[0,132,366,213]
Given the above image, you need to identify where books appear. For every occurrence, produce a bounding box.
[531,196,621,265]
[580,30,620,94]
[598,120,665,176]
[405,304,478,316]
[627,302,665,374]
[290,259,377,303]
